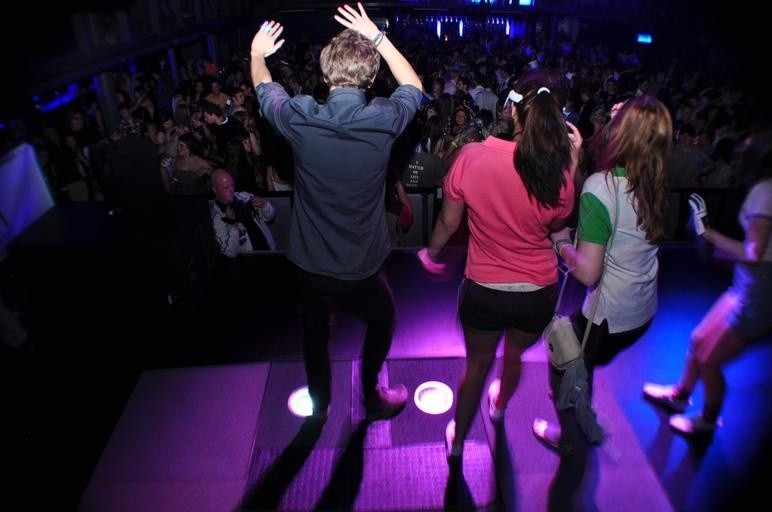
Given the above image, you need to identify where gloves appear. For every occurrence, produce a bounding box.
[688,193,710,235]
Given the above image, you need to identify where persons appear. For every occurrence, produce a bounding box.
[531,92,672,455]
[417,79,584,465]
[642,132,771,439]
[1,20,772,256]
[249,1,424,427]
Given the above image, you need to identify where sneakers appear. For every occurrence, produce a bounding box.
[644,383,714,436]
[446,419,463,461]
[368,385,408,420]
[488,379,505,421]
[534,418,561,448]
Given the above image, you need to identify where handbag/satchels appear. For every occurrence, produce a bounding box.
[542,317,581,370]
